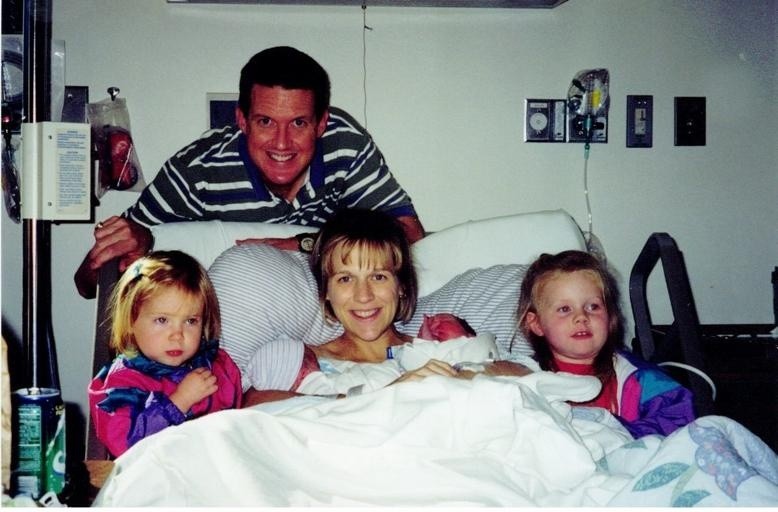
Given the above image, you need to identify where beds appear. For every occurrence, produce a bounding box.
[90,208,778,508]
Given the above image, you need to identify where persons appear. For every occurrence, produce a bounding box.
[513,249,698,439]
[241,205,535,408]
[74,45,426,301]
[417,309,478,342]
[86,248,243,457]
[246,334,323,394]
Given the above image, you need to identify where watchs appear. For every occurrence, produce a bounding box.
[294,233,317,256]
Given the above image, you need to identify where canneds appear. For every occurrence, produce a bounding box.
[10,387,67,507]
